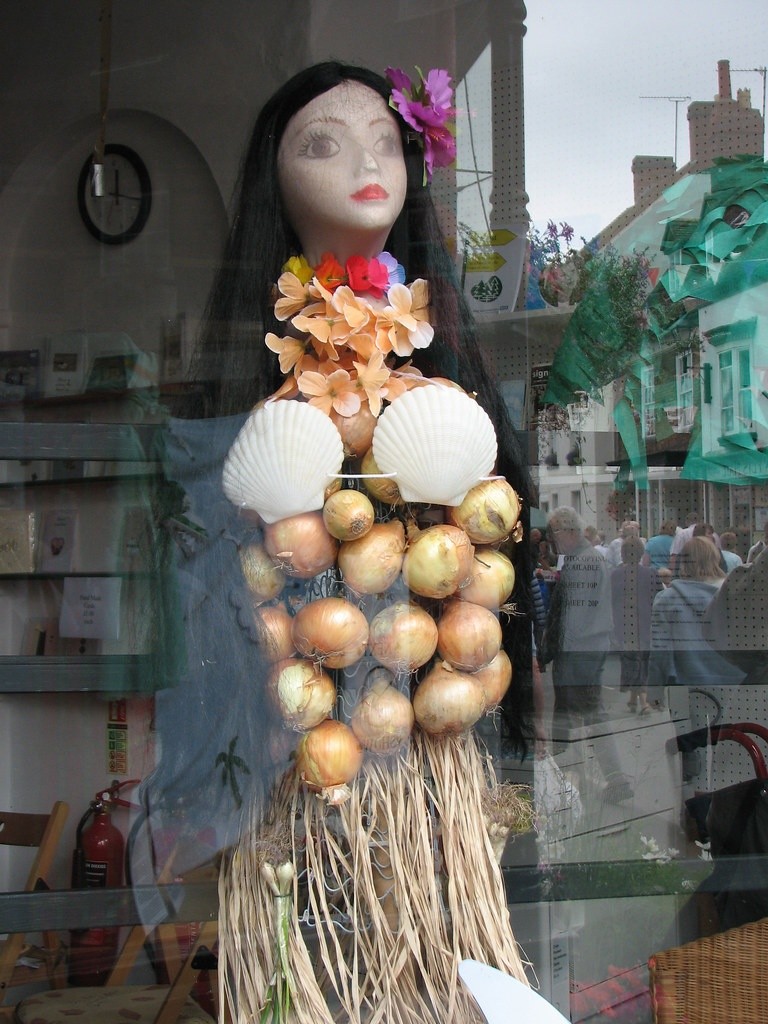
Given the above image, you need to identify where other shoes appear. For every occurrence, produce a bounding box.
[600,782,634,805]
[626,702,636,715]
[639,707,651,717]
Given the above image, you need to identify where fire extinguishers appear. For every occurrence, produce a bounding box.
[67,779,142,977]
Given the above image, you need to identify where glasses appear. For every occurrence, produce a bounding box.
[545,528,565,539]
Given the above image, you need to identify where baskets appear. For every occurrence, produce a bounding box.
[648,918,768,1024]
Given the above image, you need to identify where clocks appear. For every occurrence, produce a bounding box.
[79,144,152,246]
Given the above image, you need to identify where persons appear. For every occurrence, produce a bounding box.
[151,62,570,1023]
[528,502,767,845]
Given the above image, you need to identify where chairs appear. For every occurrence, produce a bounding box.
[15,840,219,1024]
[0,800,69,1006]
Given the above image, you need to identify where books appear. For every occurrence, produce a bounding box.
[462,222,527,317]
[0,504,156,575]
[36,330,92,400]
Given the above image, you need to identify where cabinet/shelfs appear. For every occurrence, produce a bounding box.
[0,382,207,694]
[500,708,681,1022]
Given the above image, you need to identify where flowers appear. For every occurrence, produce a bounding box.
[384,65,474,187]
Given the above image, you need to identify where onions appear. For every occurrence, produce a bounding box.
[240,378,520,785]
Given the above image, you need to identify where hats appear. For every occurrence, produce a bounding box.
[721,533,737,551]
